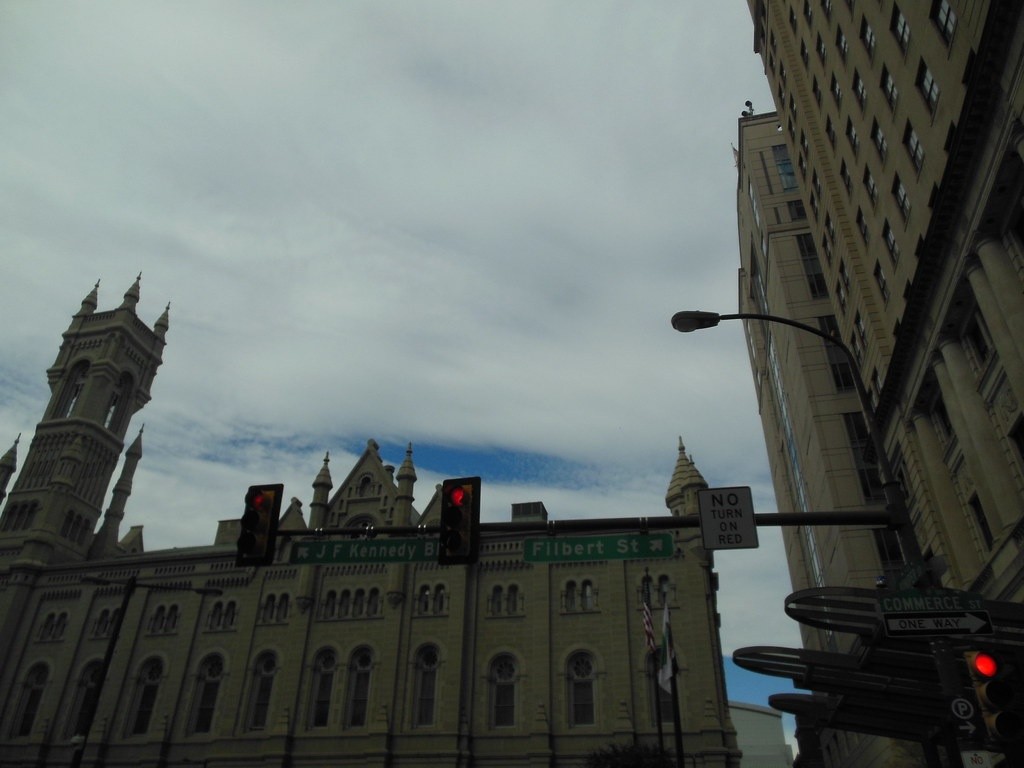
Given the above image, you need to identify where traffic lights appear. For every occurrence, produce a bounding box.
[439,474,480,566]
[963,649,1024,740]
[232,483,284,571]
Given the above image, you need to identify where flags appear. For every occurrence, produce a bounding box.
[658,601,681,695]
[642,576,658,657]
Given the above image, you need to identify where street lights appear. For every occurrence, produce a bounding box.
[672,311,965,767]
